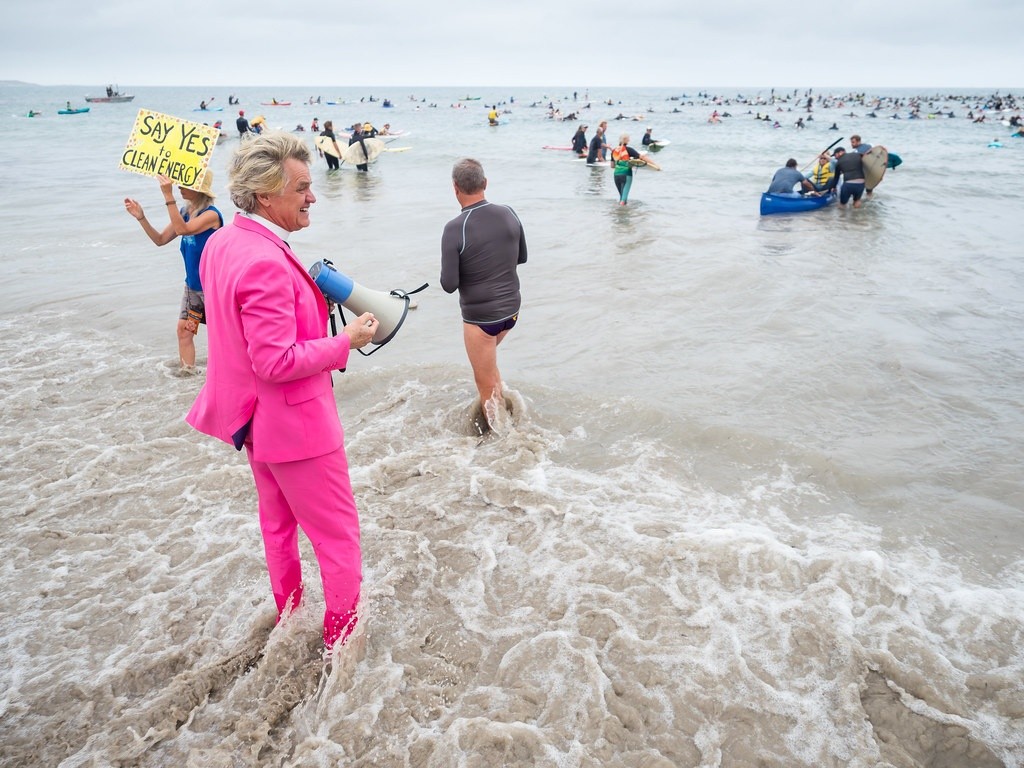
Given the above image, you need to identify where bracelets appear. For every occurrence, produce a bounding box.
[166,201,176,205]
[138,217,145,221]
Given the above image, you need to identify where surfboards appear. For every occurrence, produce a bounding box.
[648,138,671,148]
[382,146,413,153]
[312,136,348,160]
[372,128,406,138]
[542,143,612,150]
[342,138,386,165]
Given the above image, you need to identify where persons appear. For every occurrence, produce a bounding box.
[989,138,1002,147]
[488,89,655,124]
[611,135,656,205]
[203,111,268,137]
[668,88,1024,137]
[440,159,528,429]
[186,133,379,654]
[768,135,873,208]
[66,86,117,111]
[572,121,658,165]
[295,117,391,172]
[125,167,224,368]
[27,110,39,116]
[200,94,473,110]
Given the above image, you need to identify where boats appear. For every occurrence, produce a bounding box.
[58,107,89,114]
[759,171,845,216]
[192,107,223,112]
[458,97,481,100]
[327,100,360,103]
[85,96,135,103]
[261,101,291,105]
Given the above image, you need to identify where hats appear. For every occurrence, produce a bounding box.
[362,123,373,132]
[212,119,222,129]
[177,168,216,199]
[581,124,588,129]
[829,147,845,157]
[819,150,830,158]
[239,110,244,115]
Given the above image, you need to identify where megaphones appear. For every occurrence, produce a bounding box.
[308,259,409,345]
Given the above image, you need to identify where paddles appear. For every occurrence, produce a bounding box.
[799,136,846,174]
[207,97,215,106]
